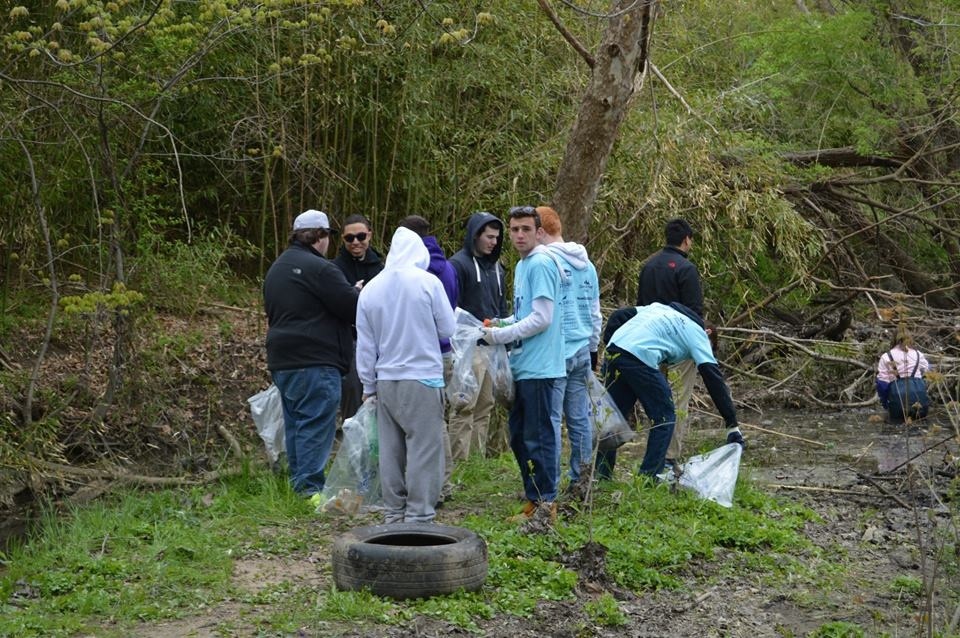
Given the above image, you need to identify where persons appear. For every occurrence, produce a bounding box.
[447,211,509,462]
[875,326,929,424]
[481,205,564,524]
[536,207,603,496]
[262,208,364,499]
[334,215,382,440]
[634,218,705,465]
[354,225,456,527]
[397,215,460,508]
[594,298,744,490]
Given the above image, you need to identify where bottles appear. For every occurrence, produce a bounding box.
[484,317,513,328]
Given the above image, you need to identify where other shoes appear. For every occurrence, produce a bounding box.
[662,467,679,484]
[652,476,664,486]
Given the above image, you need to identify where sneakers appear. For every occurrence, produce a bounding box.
[504,502,557,527]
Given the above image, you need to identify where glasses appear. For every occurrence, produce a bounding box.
[511,207,540,228]
[344,232,368,242]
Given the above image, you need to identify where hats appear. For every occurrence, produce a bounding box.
[292,209,338,235]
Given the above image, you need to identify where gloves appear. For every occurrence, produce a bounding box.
[727,428,747,449]
[479,326,494,345]
[590,351,596,370]
[599,358,616,387]
[875,380,889,409]
